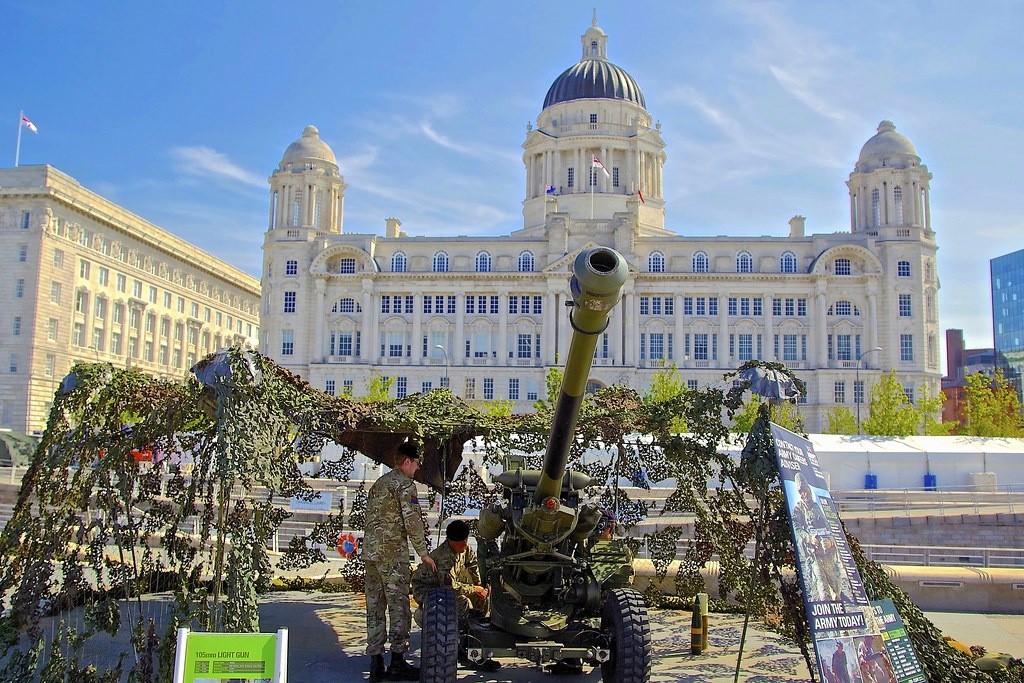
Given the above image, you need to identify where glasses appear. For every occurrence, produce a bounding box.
[405,458,420,466]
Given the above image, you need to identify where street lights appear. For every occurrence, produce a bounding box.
[435,345,449,388]
[857,345,883,435]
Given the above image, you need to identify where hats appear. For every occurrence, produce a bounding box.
[446,520,469,541]
[398,444,419,458]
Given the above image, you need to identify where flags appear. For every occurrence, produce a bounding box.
[591,155,610,178]
[638,191,645,204]
[545,185,556,194]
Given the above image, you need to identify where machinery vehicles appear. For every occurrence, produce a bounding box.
[405,245,653,683]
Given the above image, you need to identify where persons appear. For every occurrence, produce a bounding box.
[412,520,502,672]
[857,635,892,683]
[362,443,437,683]
[791,473,842,603]
[832,641,850,683]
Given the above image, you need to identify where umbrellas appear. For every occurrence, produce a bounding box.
[194,348,267,389]
[60,367,111,397]
[739,367,800,399]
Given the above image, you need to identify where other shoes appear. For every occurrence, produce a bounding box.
[387,662,421,681]
[471,660,501,670]
[369,658,385,683]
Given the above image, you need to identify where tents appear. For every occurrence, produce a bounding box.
[0,424,133,471]
